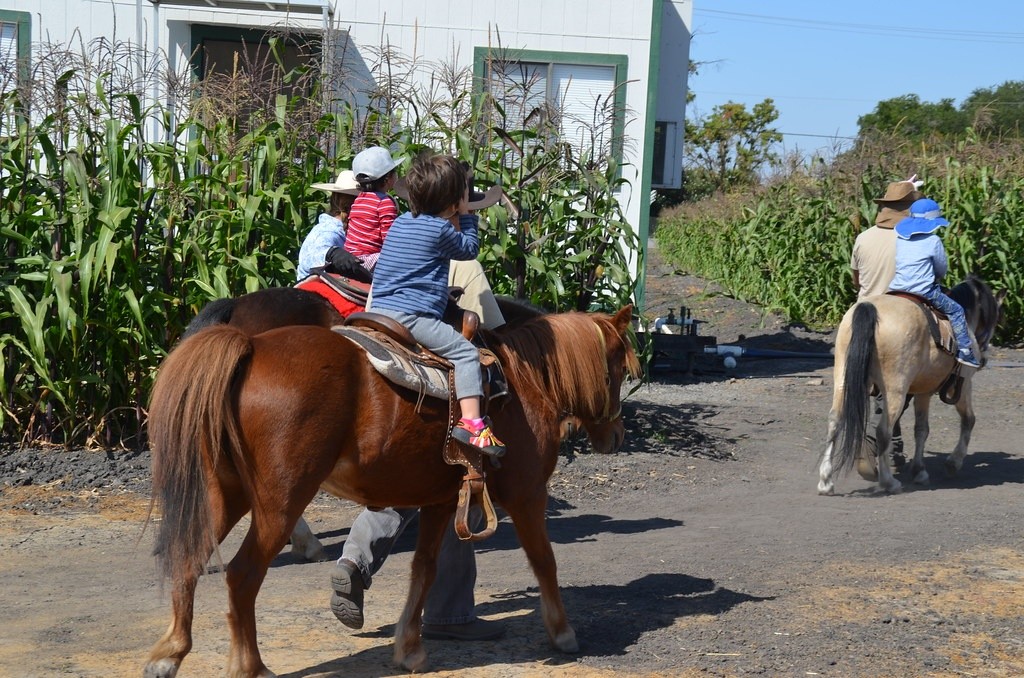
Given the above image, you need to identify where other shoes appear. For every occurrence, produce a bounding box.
[421,614,507,641]
[887,438,905,465]
[858,445,880,483]
[331,558,367,627]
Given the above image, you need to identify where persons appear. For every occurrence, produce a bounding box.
[298,144,507,639]
[889,199,980,368]
[369,154,506,457]
[851,182,926,482]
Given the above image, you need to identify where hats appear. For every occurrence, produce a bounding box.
[353,146,406,182]
[894,199,950,239]
[310,169,362,196]
[873,181,927,203]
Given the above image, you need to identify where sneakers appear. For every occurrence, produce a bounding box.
[956,347,980,368]
[452,415,506,459]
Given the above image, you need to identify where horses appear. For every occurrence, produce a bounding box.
[185,292,543,562]
[818,272,1005,494]
[139,305,637,678]
